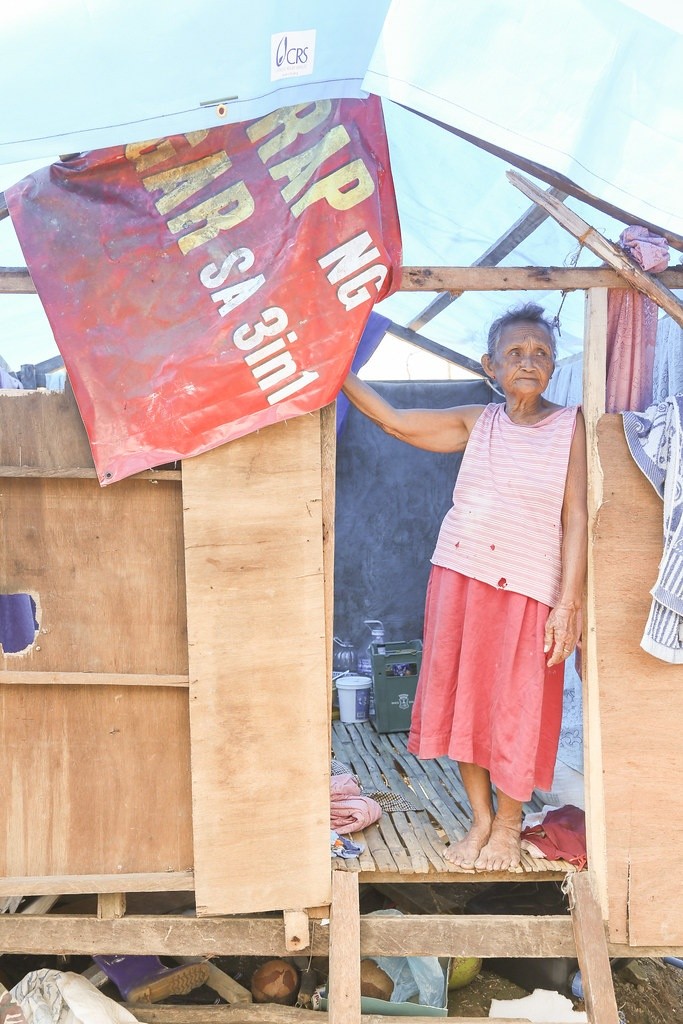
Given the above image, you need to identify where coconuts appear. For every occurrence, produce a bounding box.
[360,959,394,1001]
[250,959,299,1005]
[448,957,482,991]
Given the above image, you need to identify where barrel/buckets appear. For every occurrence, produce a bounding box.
[336,676,372,723]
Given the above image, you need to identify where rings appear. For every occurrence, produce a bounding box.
[563,649,571,653]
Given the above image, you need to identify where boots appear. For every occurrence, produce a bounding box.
[92,955,208,1003]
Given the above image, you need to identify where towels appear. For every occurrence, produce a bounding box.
[616,392,683,666]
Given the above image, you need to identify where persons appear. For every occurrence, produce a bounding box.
[337,304,588,875]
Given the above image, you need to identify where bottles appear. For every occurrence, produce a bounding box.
[332,670,349,720]
[334,637,358,673]
[358,620,385,715]
